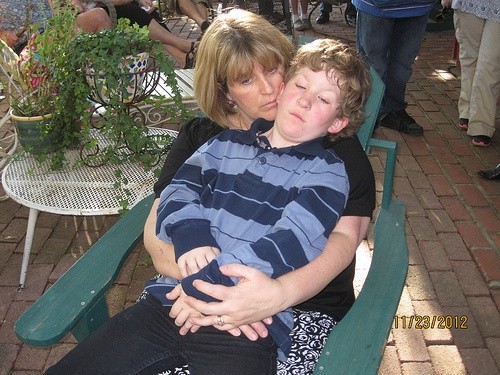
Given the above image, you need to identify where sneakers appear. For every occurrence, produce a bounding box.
[471,133,490,146]
[457,118,470,129]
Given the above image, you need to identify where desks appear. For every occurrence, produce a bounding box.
[1,128,178,289]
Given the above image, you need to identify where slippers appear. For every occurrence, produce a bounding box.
[183,50,198,69]
[188,39,201,53]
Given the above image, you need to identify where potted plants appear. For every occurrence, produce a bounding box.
[9,1,79,155]
[59,16,195,216]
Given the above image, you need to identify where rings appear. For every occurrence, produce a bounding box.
[216,315,225,327]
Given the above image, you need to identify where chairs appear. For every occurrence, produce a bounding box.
[15,36,409,375]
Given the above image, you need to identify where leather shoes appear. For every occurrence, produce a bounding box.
[477,162,500,180]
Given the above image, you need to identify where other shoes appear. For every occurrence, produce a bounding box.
[317,14,329,23]
[301,15,311,30]
[379,110,424,136]
[292,17,304,30]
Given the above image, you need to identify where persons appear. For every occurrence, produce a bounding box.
[0,0,114,57]
[68,0,202,70]
[290,0,313,31]
[350,0,440,136]
[42,36,373,375]
[316,0,357,24]
[161,0,211,32]
[134,7,377,375]
[442,0,500,147]
